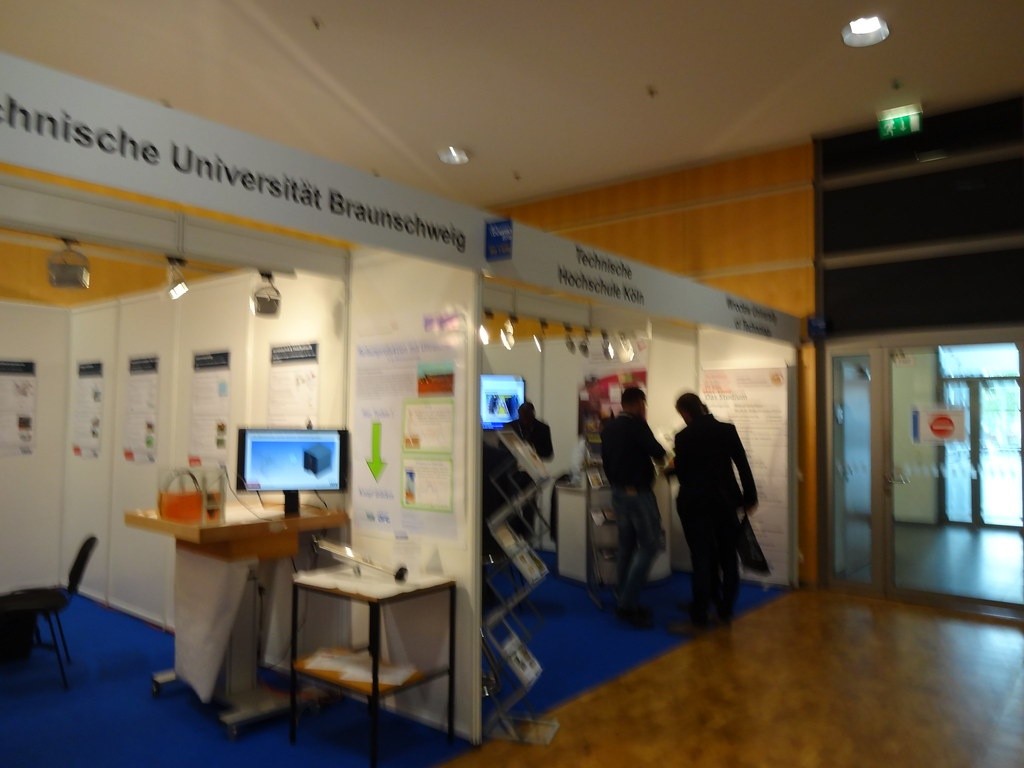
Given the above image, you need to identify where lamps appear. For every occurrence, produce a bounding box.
[479,309,634,363]
[47,234,92,290]
[164,249,191,302]
[251,267,282,319]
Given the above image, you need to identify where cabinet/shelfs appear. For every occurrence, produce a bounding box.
[287,568,456,768]
[553,472,672,585]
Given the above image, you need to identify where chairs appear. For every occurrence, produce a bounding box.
[0,534,100,690]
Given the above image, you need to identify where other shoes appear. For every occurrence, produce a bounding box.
[668,621,708,635]
[616,606,632,620]
[716,606,732,624]
[630,612,654,630]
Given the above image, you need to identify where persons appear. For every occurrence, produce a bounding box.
[601,388,666,632]
[499,401,554,549]
[664,393,759,635]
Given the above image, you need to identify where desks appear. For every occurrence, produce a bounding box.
[120,501,348,740]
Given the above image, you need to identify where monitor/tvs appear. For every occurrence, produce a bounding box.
[479,374,526,431]
[236,429,348,492]
